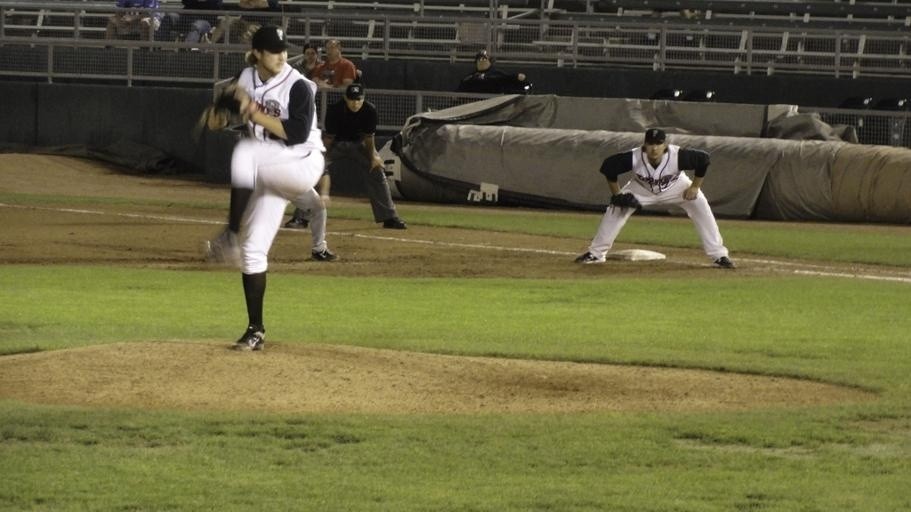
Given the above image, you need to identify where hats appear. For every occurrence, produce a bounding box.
[645,129,666,142]
[251,23,289,52]
[476,50,490,57]
[346,83,367,100]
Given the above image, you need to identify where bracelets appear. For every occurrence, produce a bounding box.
[319,194,330,200]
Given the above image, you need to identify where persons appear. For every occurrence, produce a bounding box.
[206,27,325,353]
[456,50,527,93]
[571,128,739,271]
[291,40,355,93]
[243,50,339,263]
[282,85,409,229]
[216,1,282,12]
[105,1,165,40]
[201,16,242,43]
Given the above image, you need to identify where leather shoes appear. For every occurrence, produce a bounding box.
[285,216,308,227]
[384,217,409,230]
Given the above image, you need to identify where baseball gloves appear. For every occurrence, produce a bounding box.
[208,85,251,131]
[611,192,642,212]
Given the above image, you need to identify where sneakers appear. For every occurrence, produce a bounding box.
[311,248,338,260]
[575,250,606,263]
[713,258,738,268]
[231,324,267,351]
